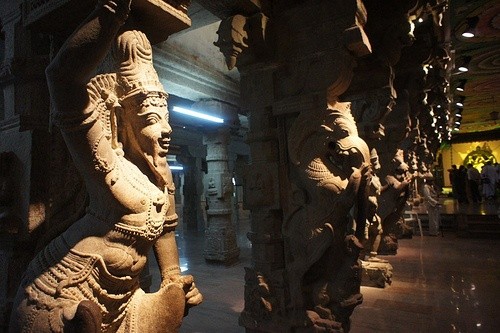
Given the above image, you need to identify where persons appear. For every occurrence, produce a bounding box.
[447,160,500,205]
[7,0,202,333]
